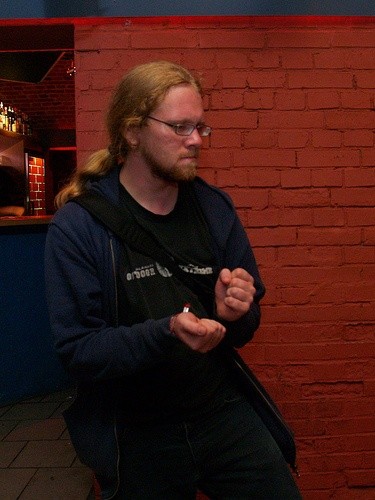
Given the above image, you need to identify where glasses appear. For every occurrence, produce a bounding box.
[148,115,212,137]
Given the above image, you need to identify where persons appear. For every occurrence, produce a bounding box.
[44,62,303,500]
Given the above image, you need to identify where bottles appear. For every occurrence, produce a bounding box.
[0,101,32,136]
